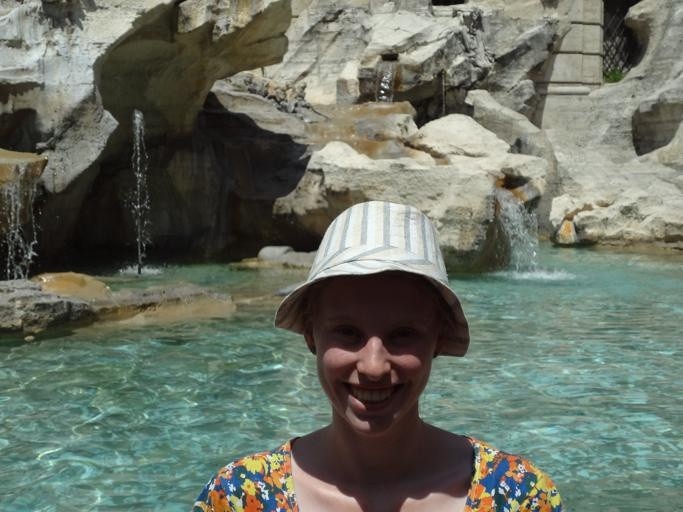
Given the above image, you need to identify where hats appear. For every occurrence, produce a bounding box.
[274,201,470,357]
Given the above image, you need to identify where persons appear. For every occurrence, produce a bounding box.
[190,200,564,512]
